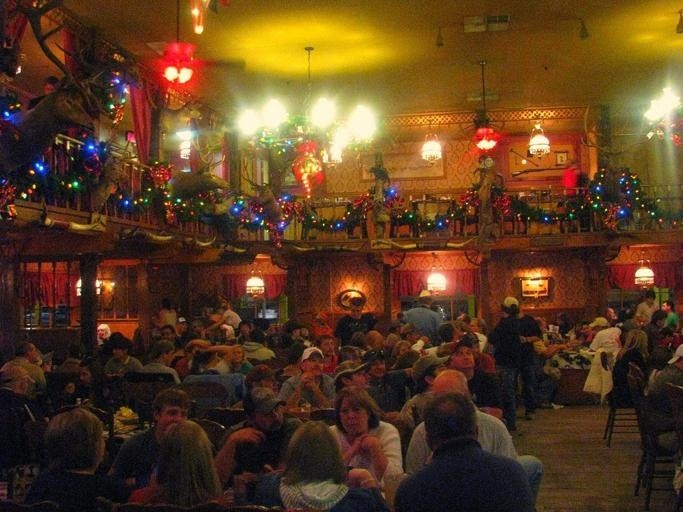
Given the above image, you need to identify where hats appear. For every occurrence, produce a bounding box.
[589,317,607,327]
[668,342,683,364]
[244,289,520,417]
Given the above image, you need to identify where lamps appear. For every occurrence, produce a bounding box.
[529,120,550,155]
[472,59,501,152]
[156,0,208,93]
[634,258,655,286]
[292,47,324,196]
[245,268,266,297]
[420,132,442,162]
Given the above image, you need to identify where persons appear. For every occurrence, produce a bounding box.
[1,290,683,512]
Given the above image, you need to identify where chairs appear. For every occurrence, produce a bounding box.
[601,348,683,512]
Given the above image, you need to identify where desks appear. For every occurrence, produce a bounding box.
[549,350,600,406]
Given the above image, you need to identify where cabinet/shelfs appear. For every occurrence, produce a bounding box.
[398,294,476,322]
